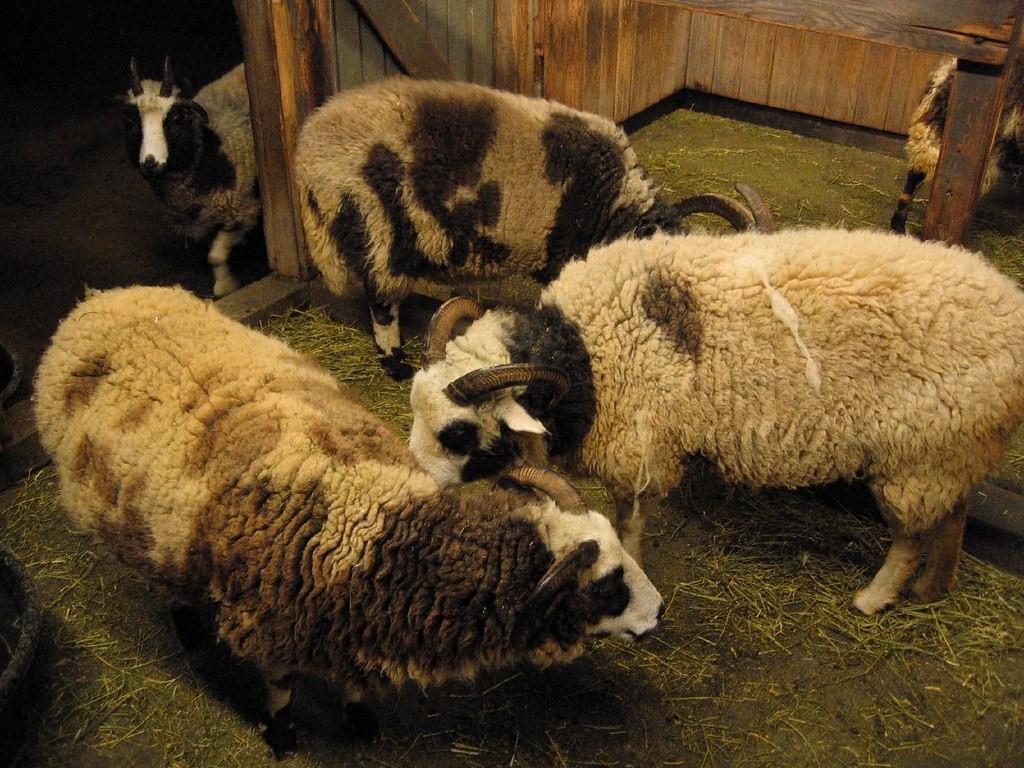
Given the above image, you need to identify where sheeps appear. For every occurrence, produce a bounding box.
[112,50,263,299]
[31,284,666,765]
[291,75,758,383]
[408,222,1023,615]
